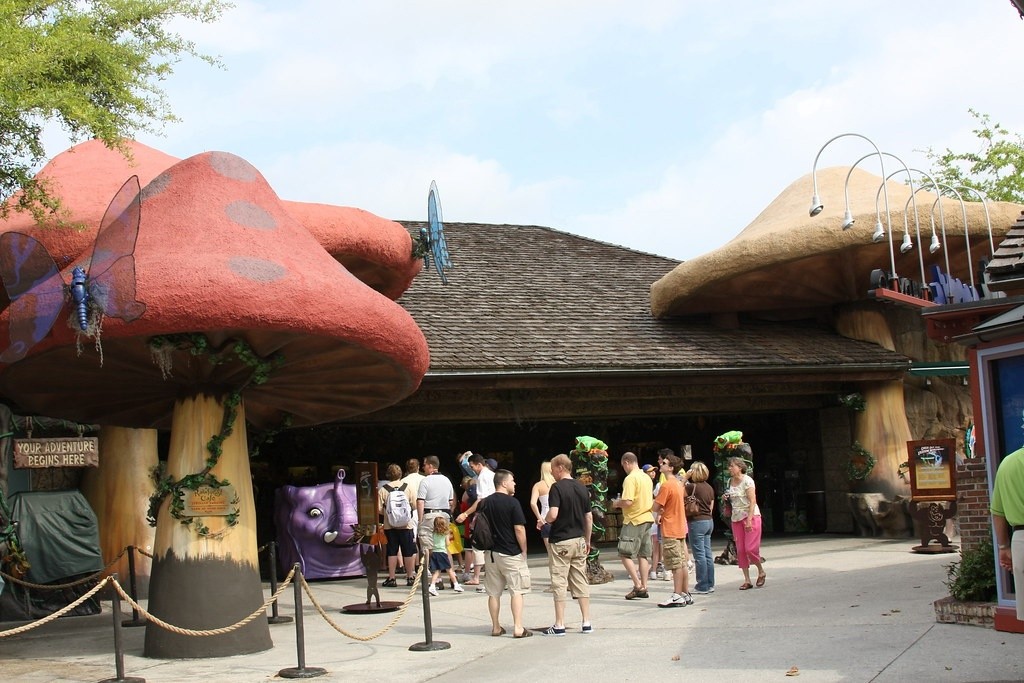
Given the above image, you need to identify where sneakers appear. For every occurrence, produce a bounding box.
[657,593,687,608]
[542,624,566,636]
[681,591,694,605]
[581,623,592,634]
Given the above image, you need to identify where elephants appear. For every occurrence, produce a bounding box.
[274,469,367,580]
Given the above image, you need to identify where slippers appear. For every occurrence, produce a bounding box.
[492,627,506,637]
[739,583,754,590]
[514,627,533,638]
[756,572,766,586]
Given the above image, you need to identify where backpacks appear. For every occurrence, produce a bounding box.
[381,483,412,528]
[470,497,494,551]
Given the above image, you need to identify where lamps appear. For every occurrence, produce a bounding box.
[808,133,899,291]
[900,182,977,303]
[873,169,955,305]
[930,186,994,260]
[841,150,929,301]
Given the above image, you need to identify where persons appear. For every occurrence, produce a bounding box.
[377,464,416,587]
[416,455,459,589]
[531,462,570,593]
[454,450,508,593]
[613,452,655,599]
[721,458,766,590]
[628,449,695,581]
[652,455,694,608]
[682,462,715,594]
[429,517,465,596]
[543,454,594,636]
[401,458,425,573]
[474,469,533,638]
[990,446,1024,621]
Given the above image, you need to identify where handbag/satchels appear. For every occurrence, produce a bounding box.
[684,483,698,515]
[724,477,731,517]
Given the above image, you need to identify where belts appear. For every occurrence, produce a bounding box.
[1014,525,1024,531]
[424,510,449,514]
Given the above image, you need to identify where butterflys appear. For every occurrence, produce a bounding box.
[411,179,453,286]
[0,174,147,363]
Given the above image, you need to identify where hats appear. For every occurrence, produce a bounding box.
[484,458,498,470]
[642,464,658,472]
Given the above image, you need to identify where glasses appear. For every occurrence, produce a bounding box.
[662,461,668,466]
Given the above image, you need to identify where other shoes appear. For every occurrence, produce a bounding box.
[625,585,649,600]
[708,586,714,593]
[625,558,695,581]
[382,560,572,596]
[688,588,708,595]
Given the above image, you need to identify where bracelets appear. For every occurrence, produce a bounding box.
[998,544,1009,549]
[464,512,467,517]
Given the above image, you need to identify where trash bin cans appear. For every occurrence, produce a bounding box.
[804,488,828,533]
